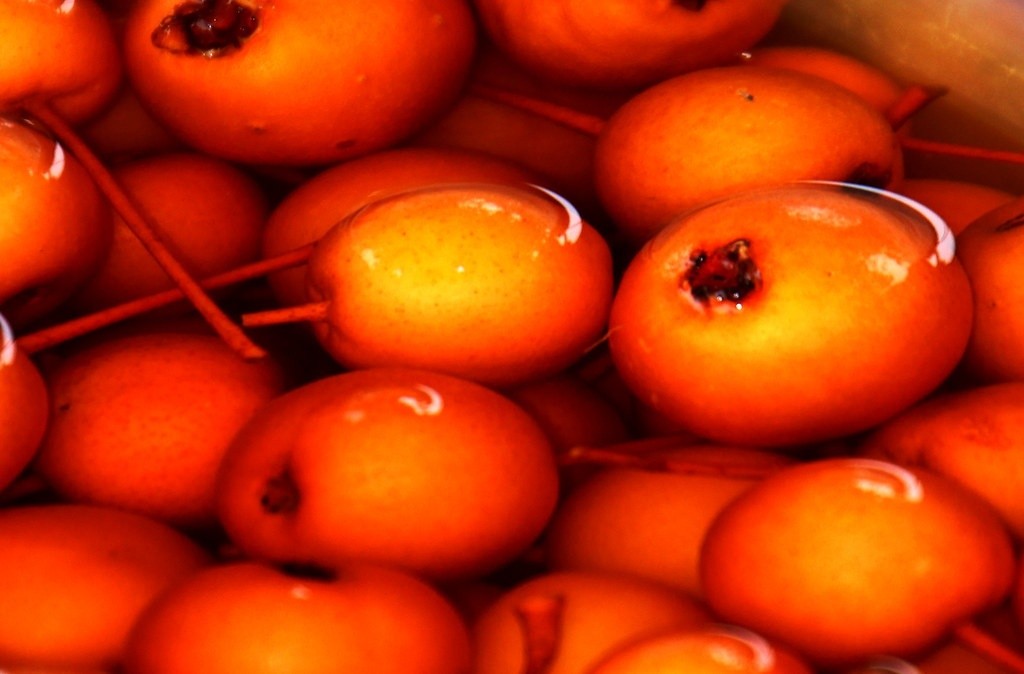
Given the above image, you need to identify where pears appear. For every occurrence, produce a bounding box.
[0,0,1024,674]
